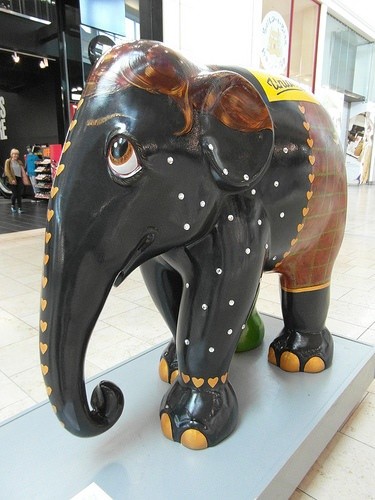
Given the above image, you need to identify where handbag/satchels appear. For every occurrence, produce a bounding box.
[2,173,9,185]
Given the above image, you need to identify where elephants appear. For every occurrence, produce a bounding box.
[38,41,347,450]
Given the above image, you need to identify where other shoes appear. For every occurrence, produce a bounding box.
[18,209,24,214]
[11,207,16,212]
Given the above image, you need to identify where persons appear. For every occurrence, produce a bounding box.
[4,149,24,213]
[26,145,42,202]
